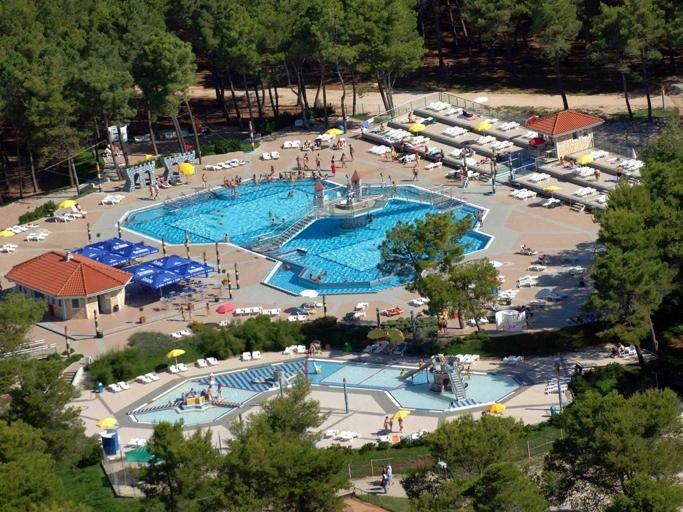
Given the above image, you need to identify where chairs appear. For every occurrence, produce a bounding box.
[108,344,305,393]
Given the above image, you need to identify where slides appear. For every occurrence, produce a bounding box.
[400,358,433,379]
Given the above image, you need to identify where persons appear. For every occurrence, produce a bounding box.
[47,110,625,495]
